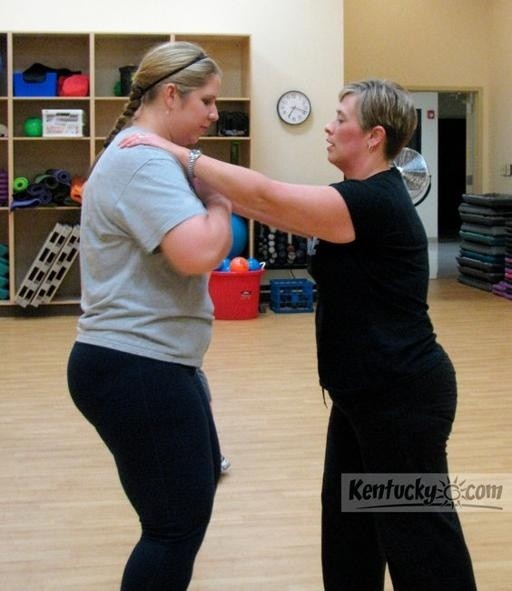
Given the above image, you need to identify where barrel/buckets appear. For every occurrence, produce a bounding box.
[208,261,267,319]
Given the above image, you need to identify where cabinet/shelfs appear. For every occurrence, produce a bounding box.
[0,30,256,308]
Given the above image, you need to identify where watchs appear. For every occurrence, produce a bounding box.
[187,147,202,179]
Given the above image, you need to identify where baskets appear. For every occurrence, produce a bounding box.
[269,280,314,313]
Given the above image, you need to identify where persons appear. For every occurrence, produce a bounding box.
[116,79,477,591]
[65,39,234,591]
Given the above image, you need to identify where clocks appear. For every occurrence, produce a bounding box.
[279,90,312,125]
